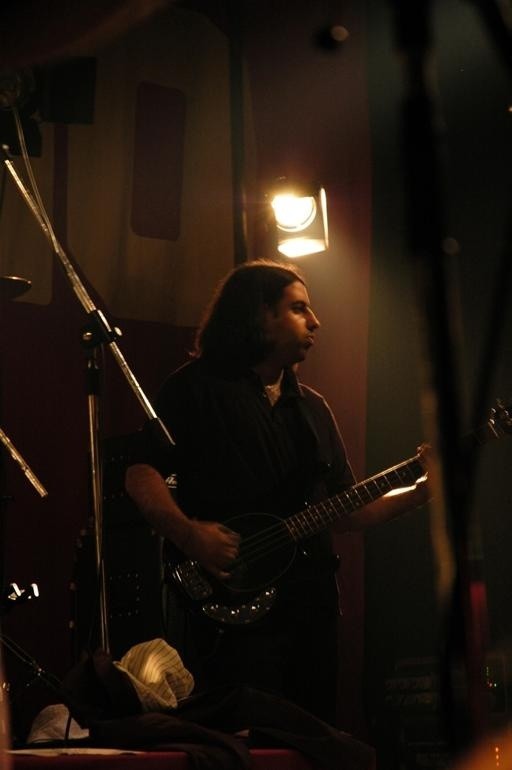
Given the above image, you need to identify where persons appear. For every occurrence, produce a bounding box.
[123,259,432,742]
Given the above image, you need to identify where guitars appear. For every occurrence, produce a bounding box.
[155,395,510,631]
